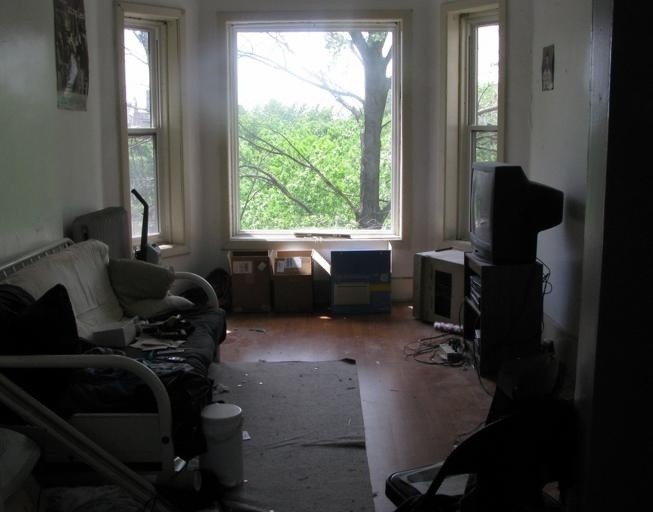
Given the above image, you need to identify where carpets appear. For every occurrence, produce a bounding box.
[193,359,387,512]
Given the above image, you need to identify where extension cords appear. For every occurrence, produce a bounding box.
[438,341,456,358]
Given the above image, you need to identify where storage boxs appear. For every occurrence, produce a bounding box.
[333,281,370,306]
[226,247,272,312]
[273,249,314,311]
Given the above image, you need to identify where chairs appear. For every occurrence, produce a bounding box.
[385,414,511,512]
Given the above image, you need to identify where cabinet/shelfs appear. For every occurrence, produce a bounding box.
[459,249,542,373]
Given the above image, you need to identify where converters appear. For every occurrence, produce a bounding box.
[447,353,463,363]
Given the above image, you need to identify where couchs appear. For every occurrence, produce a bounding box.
[0,235,227,475]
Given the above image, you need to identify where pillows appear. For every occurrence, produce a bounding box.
[105,260,194,319]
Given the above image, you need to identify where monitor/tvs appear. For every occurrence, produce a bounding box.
[466,161,565,265]
